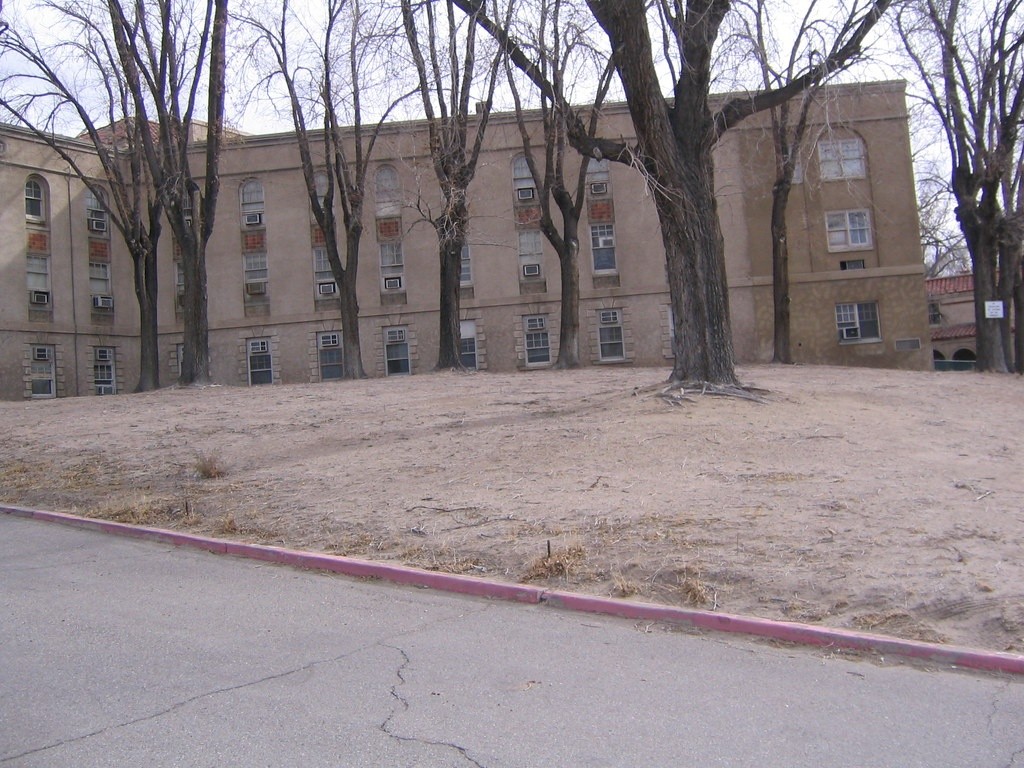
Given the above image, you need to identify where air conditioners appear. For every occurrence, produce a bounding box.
[246,214,259,224]
[320,284,333,294]
[592,183,606,193]
[525,265,539,275]
[386,279,399,288]
[247,282,264,294]
[843,327,860,339]
[93,221,106,231]
[34,294,47,303]
[101,299,112,307]
[519,189,532,199]
